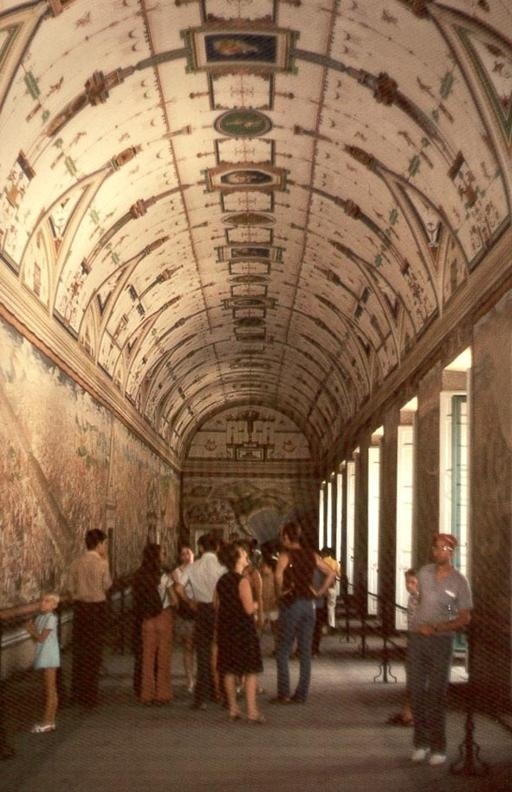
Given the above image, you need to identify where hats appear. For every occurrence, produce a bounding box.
[433,533,458,549]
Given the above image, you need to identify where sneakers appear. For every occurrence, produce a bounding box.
[429,753,447,766]
[411,747,431,761]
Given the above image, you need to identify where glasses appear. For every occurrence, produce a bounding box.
[431,544,450,553]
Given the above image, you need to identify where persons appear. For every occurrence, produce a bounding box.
[67,529,113,710]
[405,532,473,766]
[24,591,62,734]
[129,520,346,724]
[385,568,419,725]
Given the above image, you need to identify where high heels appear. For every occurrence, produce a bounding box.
[229,708,246,722]
[247,711,266,725]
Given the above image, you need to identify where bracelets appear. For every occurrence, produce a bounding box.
[427,623,437,632]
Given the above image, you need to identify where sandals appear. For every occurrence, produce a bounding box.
[31,724,52,734]
[51,723,56,730]
